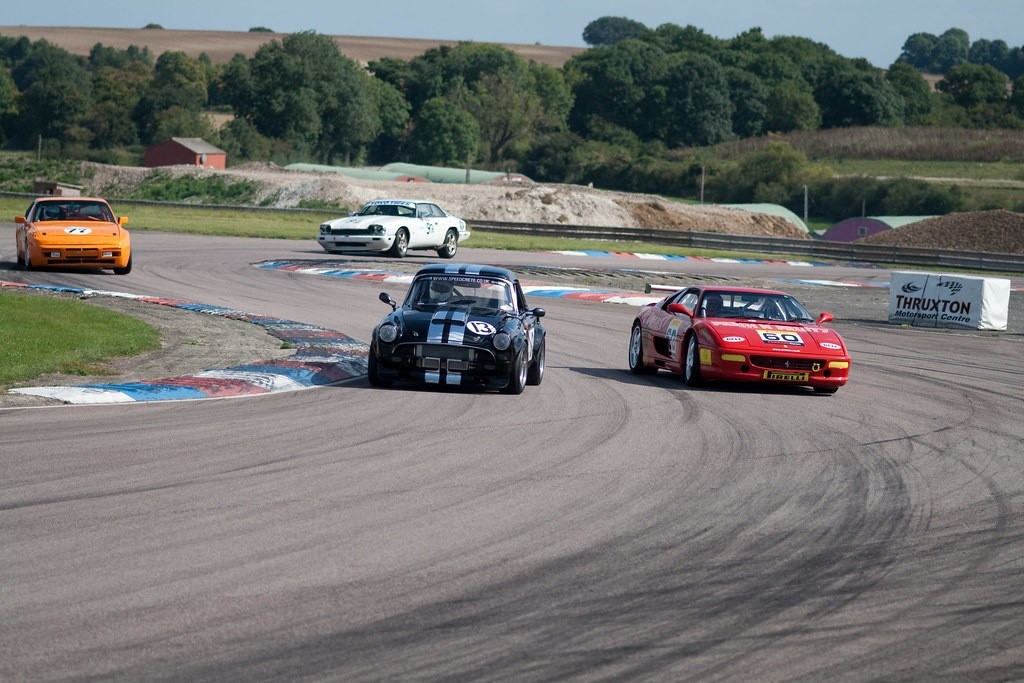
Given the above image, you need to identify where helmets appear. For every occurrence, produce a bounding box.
[429,280,452,302]
[706,295,723,311]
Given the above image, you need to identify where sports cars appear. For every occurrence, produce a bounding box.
[314,199,472,258]
[628,286,852,396]
[14,196,133,275]
[368,262,548,395]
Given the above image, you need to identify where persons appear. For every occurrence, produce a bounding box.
[707,295,723,315]
[43,206,60,219]
[429,281,453,302]
